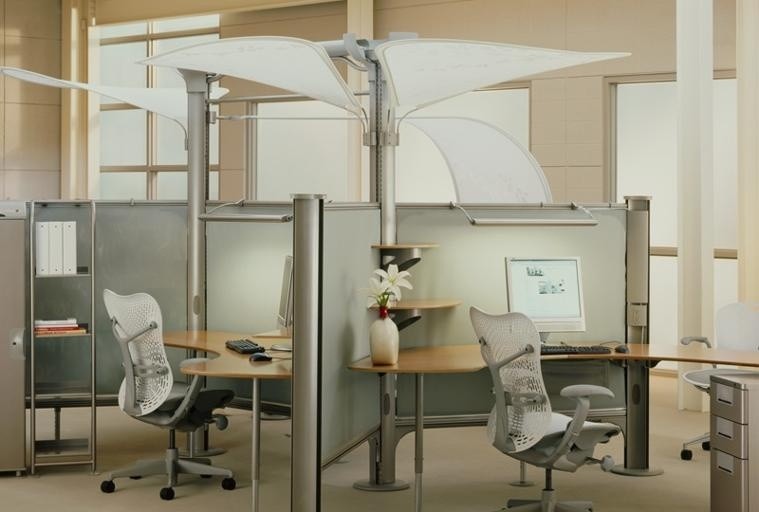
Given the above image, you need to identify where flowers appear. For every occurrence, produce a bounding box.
[366,263,411,320]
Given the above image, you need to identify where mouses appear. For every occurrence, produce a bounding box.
[614,344,628,353]
[249,353,273,362]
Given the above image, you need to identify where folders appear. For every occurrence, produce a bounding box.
[35,221,77,276]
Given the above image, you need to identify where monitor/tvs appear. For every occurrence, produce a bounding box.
[271,255,294,352]
[505,256,587,345]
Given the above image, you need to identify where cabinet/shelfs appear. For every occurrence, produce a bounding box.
[364,233,461,315]
[23,199,102,477]
[705,372,759,512]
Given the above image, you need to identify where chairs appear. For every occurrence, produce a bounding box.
[469,302,624,512]
[671,300,759,461]
[85,287,243,500]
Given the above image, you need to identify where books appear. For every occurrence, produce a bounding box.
[35,318,87,336]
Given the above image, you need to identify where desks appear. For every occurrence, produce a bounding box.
[153,321,294,512]
[343,336,758,499]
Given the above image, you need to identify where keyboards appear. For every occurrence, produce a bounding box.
[542,345,611,355]
[225,337,266,354]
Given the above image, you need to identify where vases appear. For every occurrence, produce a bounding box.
[369,314,400,366]
